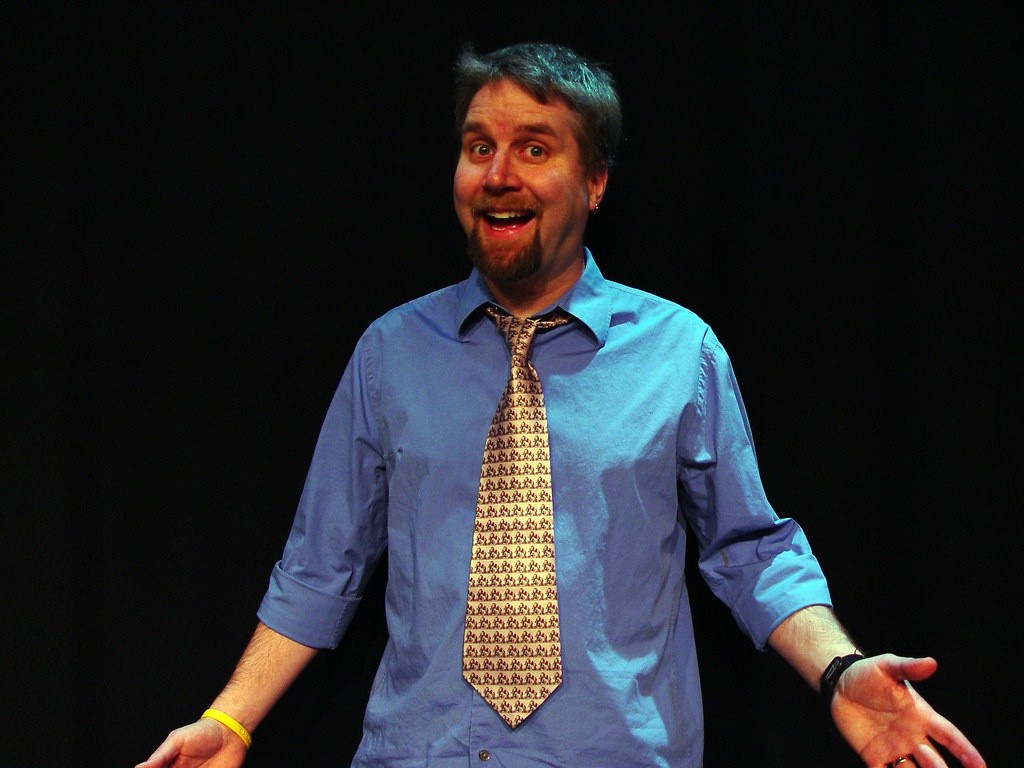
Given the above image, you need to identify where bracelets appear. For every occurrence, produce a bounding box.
[201,709,251,750]
[818,653,866,710]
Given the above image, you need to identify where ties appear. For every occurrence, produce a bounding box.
[460,304,570,728]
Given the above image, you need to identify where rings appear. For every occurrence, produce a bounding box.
[894,757,911,768]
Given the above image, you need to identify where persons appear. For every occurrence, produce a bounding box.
[139,42,987,768]
